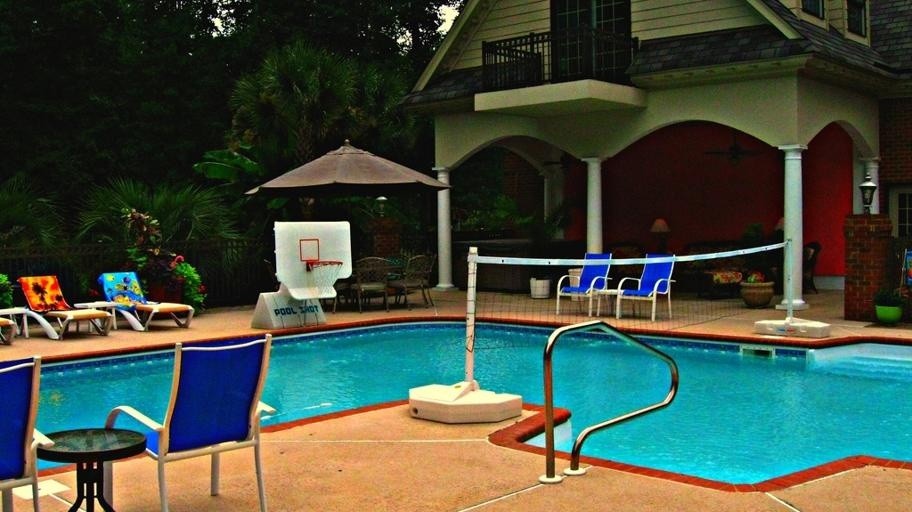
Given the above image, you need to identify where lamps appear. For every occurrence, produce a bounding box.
[650,218,672,232]
[859,173,877,213]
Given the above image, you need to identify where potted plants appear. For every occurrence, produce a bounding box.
[874,289,906,325]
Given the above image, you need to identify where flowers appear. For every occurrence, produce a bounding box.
[123,209,214,315]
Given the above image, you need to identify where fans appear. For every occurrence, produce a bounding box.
[703,136,768,166]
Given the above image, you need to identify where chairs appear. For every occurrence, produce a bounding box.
[556,239,821,322]
[0,272,196,344]
[322,254,437,313]
[104,333,277,512]
[0,355,43,512]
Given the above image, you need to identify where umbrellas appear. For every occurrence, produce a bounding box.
[244,139,452,220]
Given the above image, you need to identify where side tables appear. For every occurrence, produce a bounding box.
[36,428,147,512]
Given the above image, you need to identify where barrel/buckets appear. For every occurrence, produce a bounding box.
[568,268,584,301]
